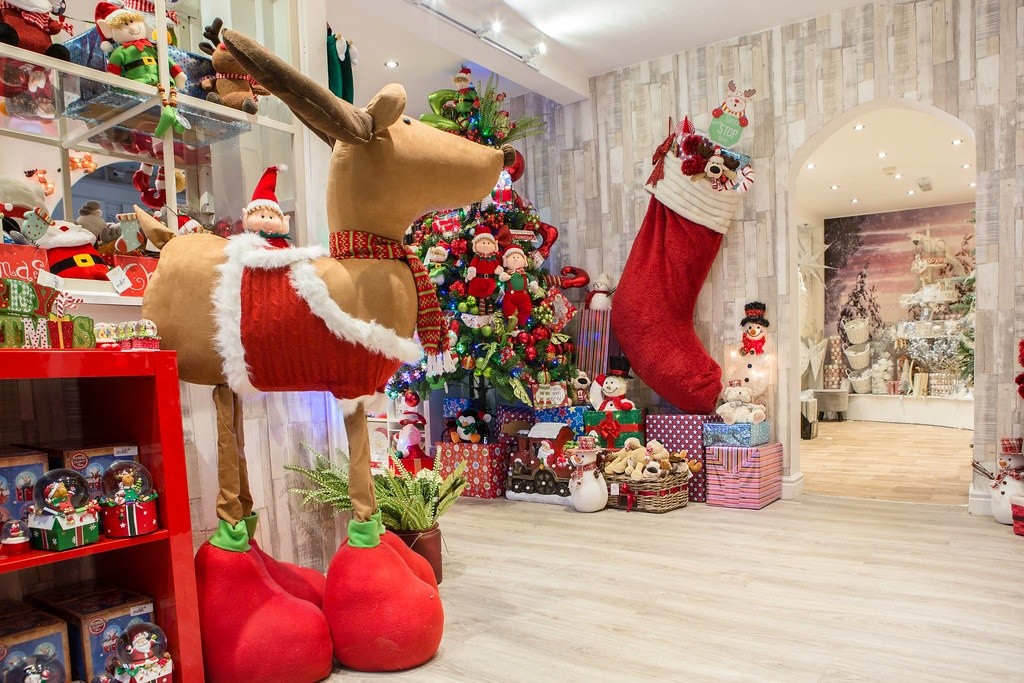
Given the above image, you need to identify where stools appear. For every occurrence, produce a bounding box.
[812,390,849,422]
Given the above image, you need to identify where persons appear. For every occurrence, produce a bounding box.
[499,245,538,329]
[125,625,161,669]
[452,68,480,118]
[95,3,187,137]
[468,225,504,316]
[427,240,450,287]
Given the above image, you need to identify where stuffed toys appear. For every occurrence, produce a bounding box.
[569,369,702,512]
[610,132,755,414]
[113,0,182,45]
[142,27,503,683]
[990,437,1024,524]
[0,122,291,280]
[445,407,490,444]
[198,16,272,114]
[585,273,612,310]
[716,379,767,425]
[0,0,73,124]
[394,409,427,459]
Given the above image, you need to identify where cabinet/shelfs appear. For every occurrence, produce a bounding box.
[0,346,205,683]
[366,390,432,474]
[0,0,307,309]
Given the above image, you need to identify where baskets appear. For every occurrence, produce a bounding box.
[602,470,689,514]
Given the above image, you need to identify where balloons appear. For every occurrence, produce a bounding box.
[526,220,559,261]
[420,90,460,131]
[542,266,589,289]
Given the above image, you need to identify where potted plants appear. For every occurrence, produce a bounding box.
[282,440,467,585]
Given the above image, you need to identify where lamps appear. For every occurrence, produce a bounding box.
[475,19,501,39]
[523,44,547,62]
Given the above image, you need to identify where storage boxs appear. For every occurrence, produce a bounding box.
[0,444,140,532]
[801,399,817,440]
[885,372,928,396]
[0,581,161,683]
[390,285,782,513]
